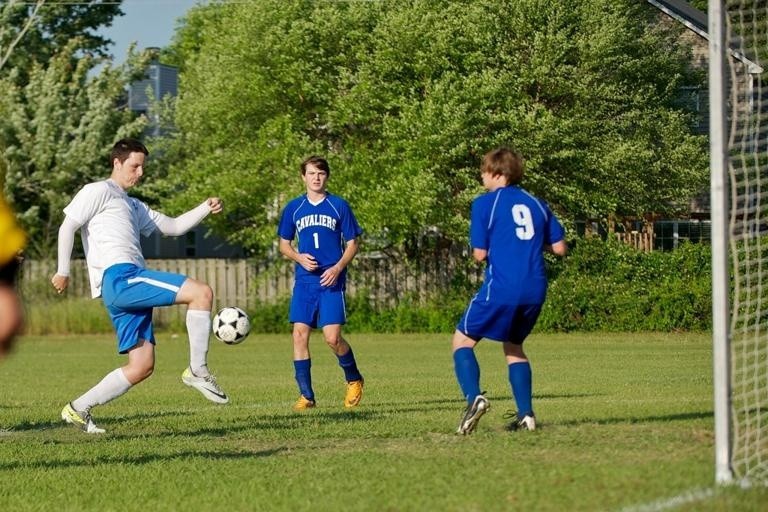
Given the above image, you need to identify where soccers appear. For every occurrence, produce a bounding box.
[213,306,252,346]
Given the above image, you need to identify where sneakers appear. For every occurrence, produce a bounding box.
[343,375,365,408]
[456,395,492,436]
[61,401,107,435]
[504,414,537,432]
[291,394,316,412]
[180,365,230,404]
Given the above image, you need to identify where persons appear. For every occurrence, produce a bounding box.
[49,138,229,433]
[276,154,365,410]
[451,147,567,435]
